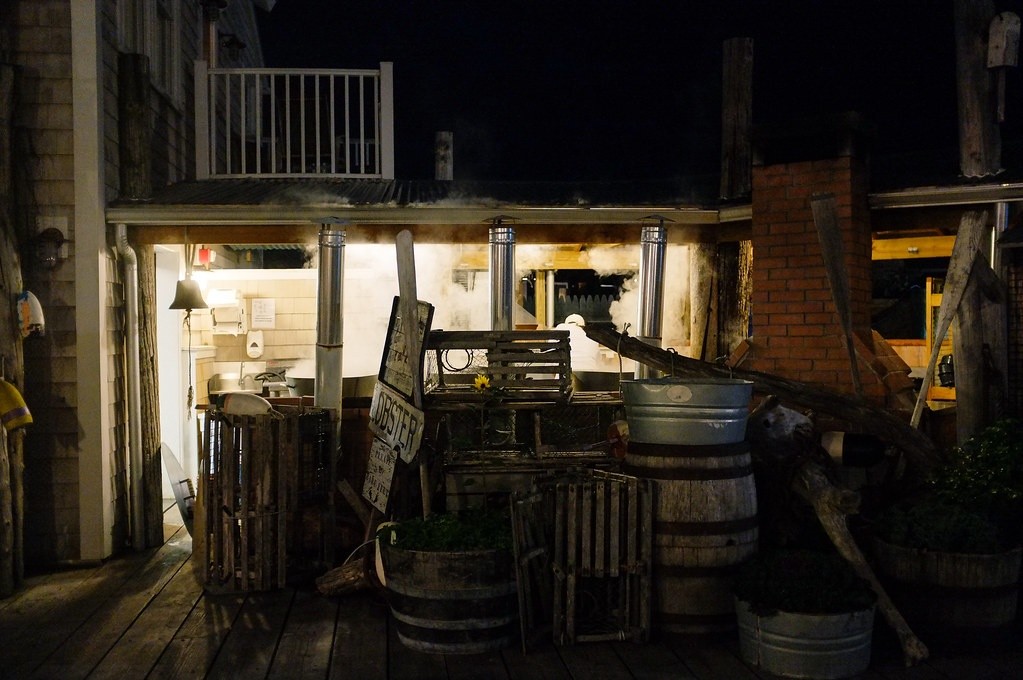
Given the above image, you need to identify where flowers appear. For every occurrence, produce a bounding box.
[474,375,508,523]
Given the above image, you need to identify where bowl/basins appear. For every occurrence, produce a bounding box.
[620,378,754,445]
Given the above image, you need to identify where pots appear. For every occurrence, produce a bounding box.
[573,371,634,392]
[280,374,378,398]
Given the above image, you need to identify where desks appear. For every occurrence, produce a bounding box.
[441,459,612,513]
[245,134,375,172]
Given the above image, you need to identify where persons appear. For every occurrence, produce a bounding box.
[548,314,604,386]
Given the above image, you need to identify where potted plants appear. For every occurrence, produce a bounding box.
[733,549,884,680]
[869,418,1023,654]
[375,509,515,655]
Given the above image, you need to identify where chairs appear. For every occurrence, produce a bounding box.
[276,94,340,173]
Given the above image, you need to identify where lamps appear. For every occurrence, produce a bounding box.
[218,29,247,62]
